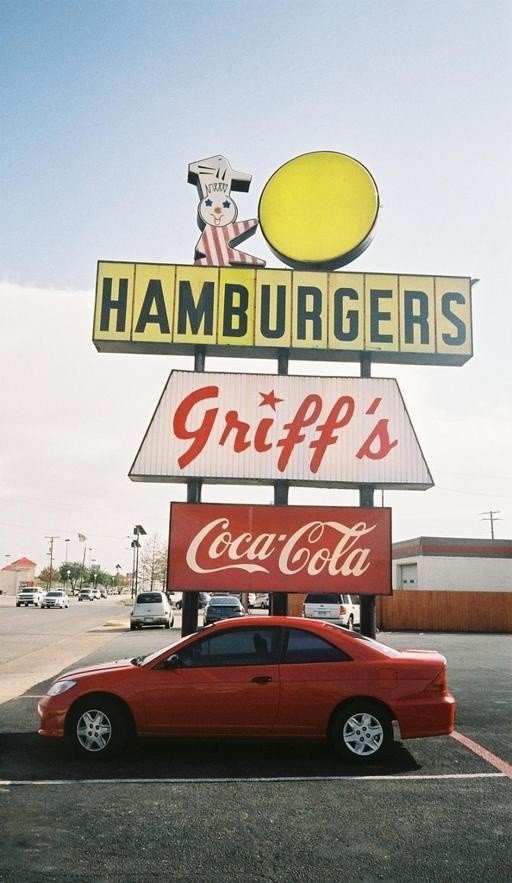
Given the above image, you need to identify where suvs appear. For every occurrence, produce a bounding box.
[303,593,360,631]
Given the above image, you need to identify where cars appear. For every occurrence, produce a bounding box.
[37,617,456,762]
[169,590,269,627]
[16,587,106,609]
[129,590,174,628]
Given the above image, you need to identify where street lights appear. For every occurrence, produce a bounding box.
[94,574,97,589]
[112,577,114,586]
[130,524,146,597]
[116,564,122,574]
[66,570,70,594]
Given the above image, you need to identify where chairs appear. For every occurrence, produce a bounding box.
[248,633,267,664]
